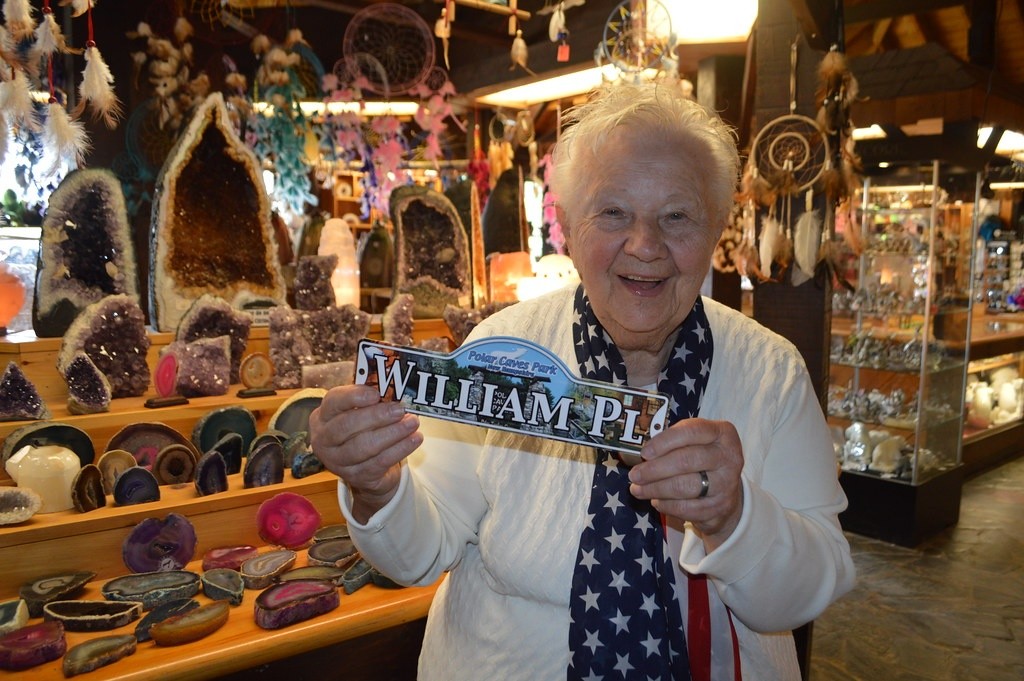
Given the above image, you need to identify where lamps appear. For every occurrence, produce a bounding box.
[469,58,667,112]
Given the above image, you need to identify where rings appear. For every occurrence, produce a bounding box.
[696,471,709,499]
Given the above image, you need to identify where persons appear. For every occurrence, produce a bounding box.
[308,80,854,680]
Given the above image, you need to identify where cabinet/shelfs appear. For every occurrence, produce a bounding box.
[0,316,458,681]
[320,170,443,248]
[826,135,1024,550]
